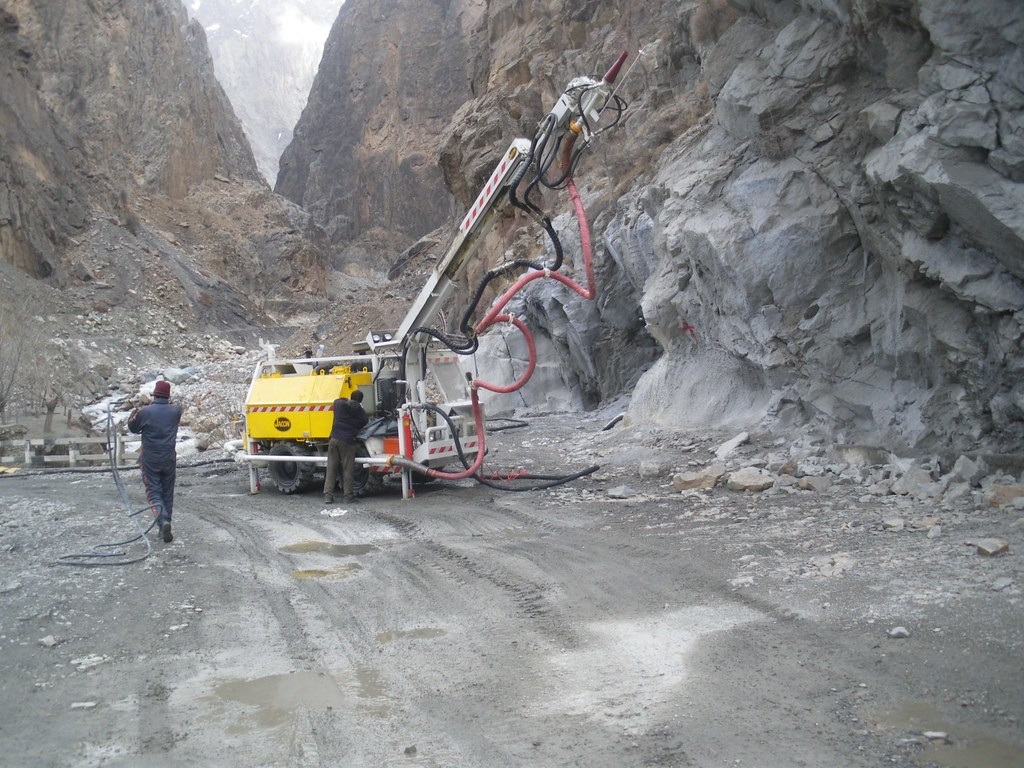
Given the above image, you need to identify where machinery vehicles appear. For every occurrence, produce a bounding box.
[243,49,639,500]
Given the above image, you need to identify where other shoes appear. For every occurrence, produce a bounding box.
[342,496,360,505]
[157,519,173,543]
[324,494,334,503]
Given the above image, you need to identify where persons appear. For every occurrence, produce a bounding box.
[128,381,182,543]
[323,391,368,504]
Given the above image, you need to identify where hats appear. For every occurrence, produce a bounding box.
[149,381,171,399]
